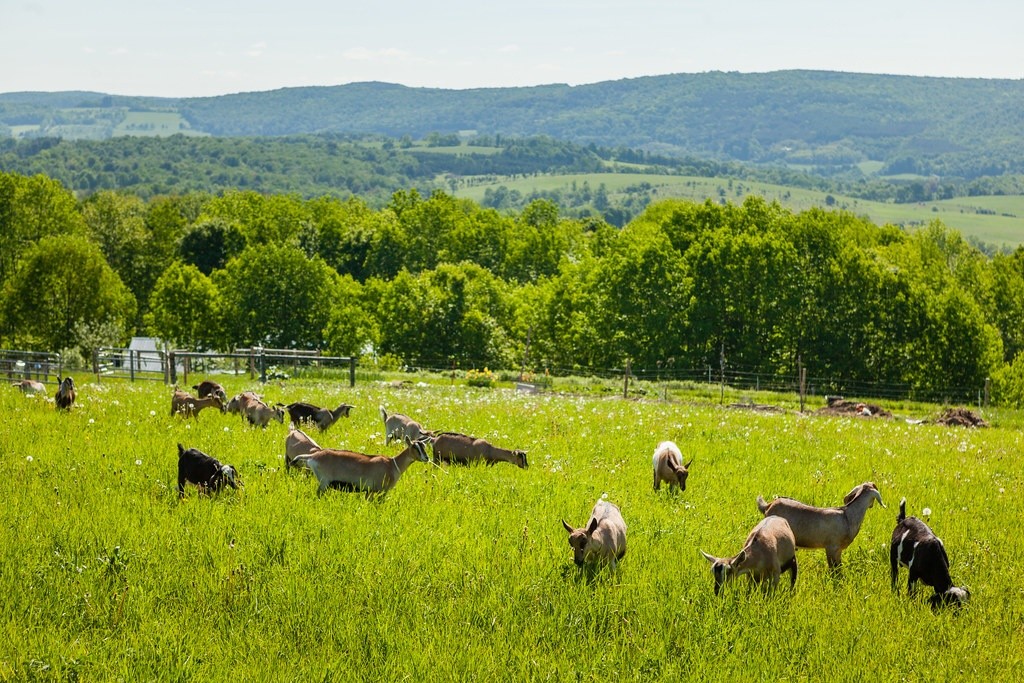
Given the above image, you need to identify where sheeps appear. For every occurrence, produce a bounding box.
[755,482,886,570]
[651,440,694,497]
[887,497,967,617]
[699,514,798,597]
[169,378,531,507]
[10,374,77,412]
[561,498,628,584]
